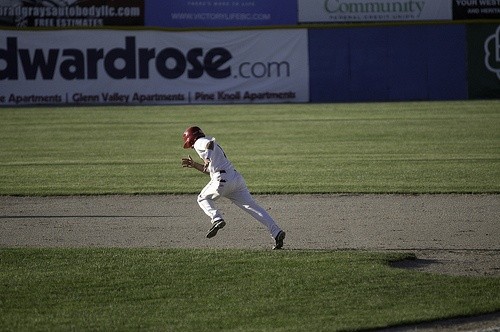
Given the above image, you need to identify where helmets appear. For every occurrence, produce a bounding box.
[183,126,205,148]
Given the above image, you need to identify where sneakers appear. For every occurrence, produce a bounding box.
[206,220,226,238]
[272,231,285,250]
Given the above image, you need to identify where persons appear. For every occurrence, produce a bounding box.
[182,125,286,250]
